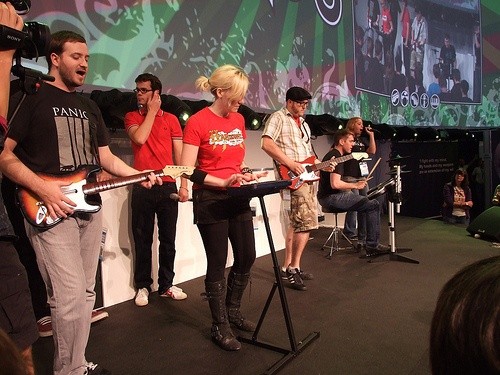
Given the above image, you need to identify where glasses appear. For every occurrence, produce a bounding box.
[294,101,308,107]
[133,88,152,94]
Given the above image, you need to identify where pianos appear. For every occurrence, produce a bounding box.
[215,179,321,375]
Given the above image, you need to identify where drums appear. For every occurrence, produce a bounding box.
[367,178,395,201]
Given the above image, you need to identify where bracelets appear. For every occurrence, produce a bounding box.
[180,187,189,192]
[240,167,252,175]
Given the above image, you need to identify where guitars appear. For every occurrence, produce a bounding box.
[16,164,193,230]
[279,152,368,189]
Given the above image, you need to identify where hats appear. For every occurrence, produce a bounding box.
[286,87,312,101]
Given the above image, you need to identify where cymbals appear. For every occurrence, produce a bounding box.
[386,154,410,175]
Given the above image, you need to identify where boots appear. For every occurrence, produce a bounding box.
[226,267,257,333]
[200,278,241,351]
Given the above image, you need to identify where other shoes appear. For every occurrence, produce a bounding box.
[366,245,396,256]
[357,241,366,245]
[37,316,53,337]
[342,236,358,240]
[91,310,109,324]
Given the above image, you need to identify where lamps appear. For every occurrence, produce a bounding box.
[324,114,344,132]
[404,126,419,139]
[90,89,134,118]
[430,128,441,140]
[161,94,192,126]
[238,105,261,130]
[382,123,397,137]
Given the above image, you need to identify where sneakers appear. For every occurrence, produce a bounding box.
[135,288,149,306]
[281,267,313,280]
[160,286,187,300]
[286,263,306,291]
[84,361,113,375]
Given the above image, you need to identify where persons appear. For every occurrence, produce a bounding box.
[441,168,474,226]
[260,87,337,291]
[355,0,481,103]
[181,65,269,351]
[317,130,391,255]
[329,117,376,244]
[1,176,109,336]
[0,30,162,375]
[0,2,41,375]
[429,256,500,375]
[123,73,189,307]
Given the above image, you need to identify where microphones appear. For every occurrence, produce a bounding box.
[364,127,380,132]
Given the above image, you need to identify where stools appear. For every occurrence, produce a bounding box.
[321,207,360,259]
